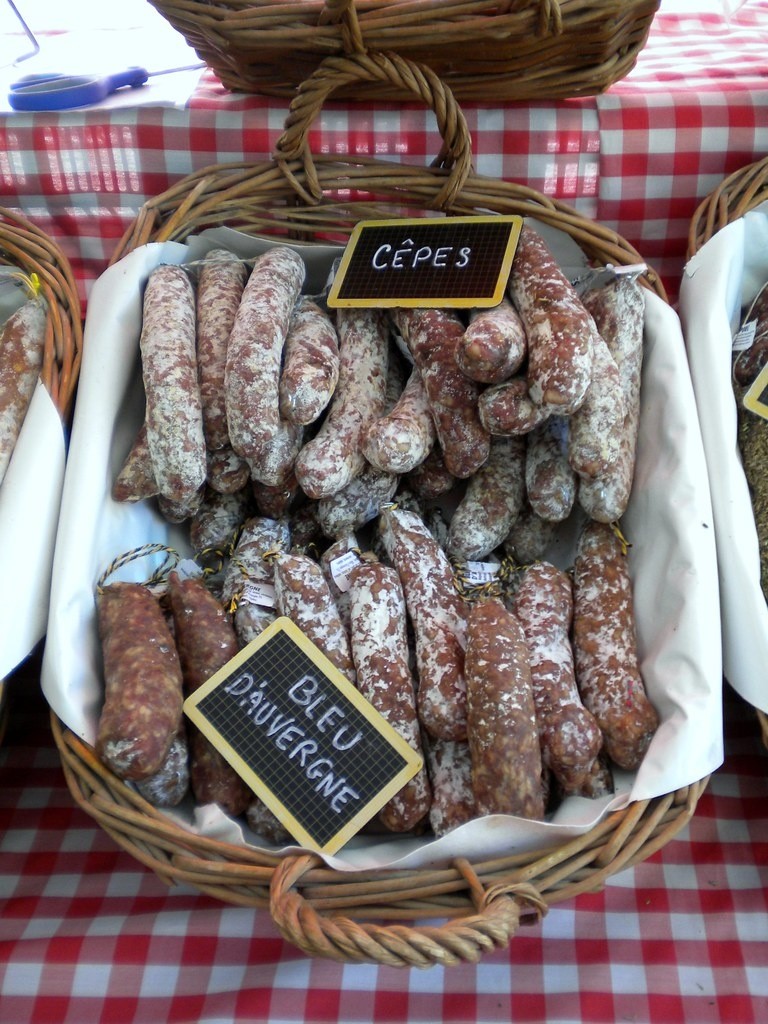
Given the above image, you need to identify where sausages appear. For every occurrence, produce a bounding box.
[100,226,655,845]
[0,298,50,490]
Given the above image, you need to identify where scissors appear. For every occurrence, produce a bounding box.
[8,60,207,112]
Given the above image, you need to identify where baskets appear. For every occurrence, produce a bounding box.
[685,153,768,742]
[48,51,726,970]
[147,0,663,99]
[0,201,82,419]
